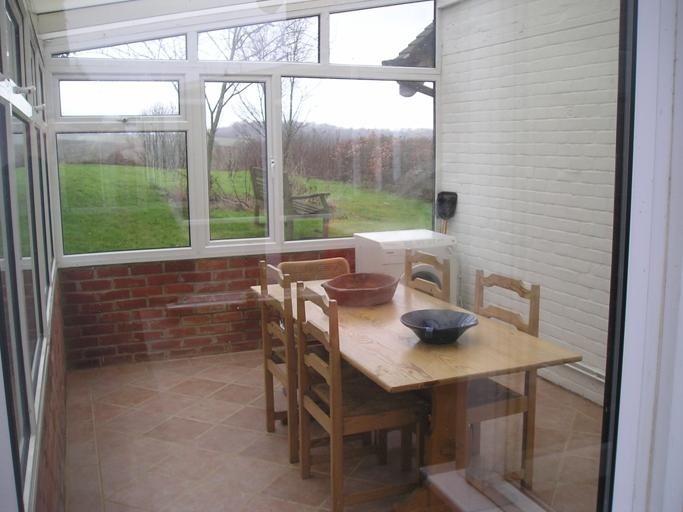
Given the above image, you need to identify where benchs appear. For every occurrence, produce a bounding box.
[248,163,339,239]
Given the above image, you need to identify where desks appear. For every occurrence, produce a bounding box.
[250,279,584,512]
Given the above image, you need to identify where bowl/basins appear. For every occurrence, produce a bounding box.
[321,271,401,308]
[397,310,478,347]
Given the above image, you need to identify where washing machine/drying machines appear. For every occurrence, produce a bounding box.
[353,228,459,307]
